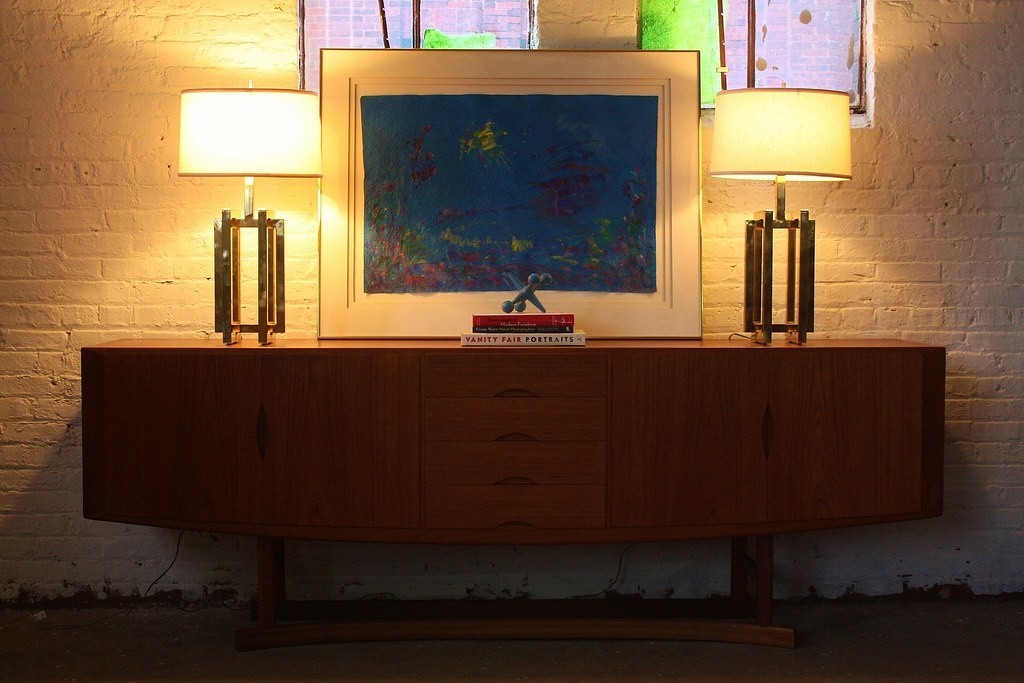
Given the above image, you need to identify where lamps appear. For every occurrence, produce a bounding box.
[177,88,322,344]
[708,88,852,343]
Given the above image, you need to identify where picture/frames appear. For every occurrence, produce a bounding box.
[319,47,702,340]
[636,0,868,113]
[298,0,534,92]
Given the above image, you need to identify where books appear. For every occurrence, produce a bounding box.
[461,329,586,345]
[472,326,574,333]
[472,314,574,326]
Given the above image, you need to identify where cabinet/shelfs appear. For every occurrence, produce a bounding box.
[81,338,945,651]
